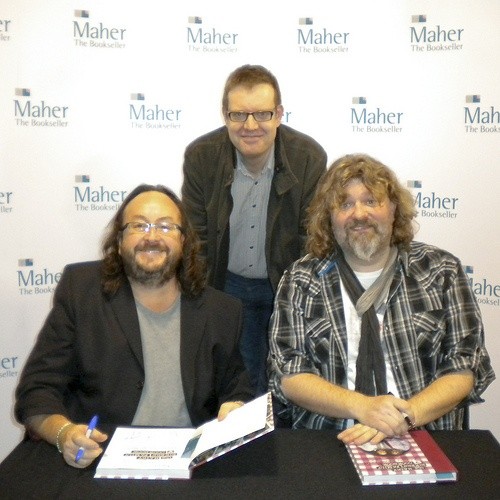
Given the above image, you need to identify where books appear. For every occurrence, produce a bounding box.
[340,430,459,485]
[95,392,276,481]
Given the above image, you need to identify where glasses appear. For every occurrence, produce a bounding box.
[226,106,277,122]
[119,222,184,233]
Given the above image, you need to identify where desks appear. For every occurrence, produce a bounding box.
[0,429,500,500]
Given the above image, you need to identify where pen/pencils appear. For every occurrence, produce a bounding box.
[388,392,413,428]
[74,416,98,462]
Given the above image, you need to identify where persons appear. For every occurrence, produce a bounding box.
[263,153,497,447]
[178,63,328,397]
[12,183,256,469]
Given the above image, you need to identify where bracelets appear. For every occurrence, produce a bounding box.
[56,422,71,453]
